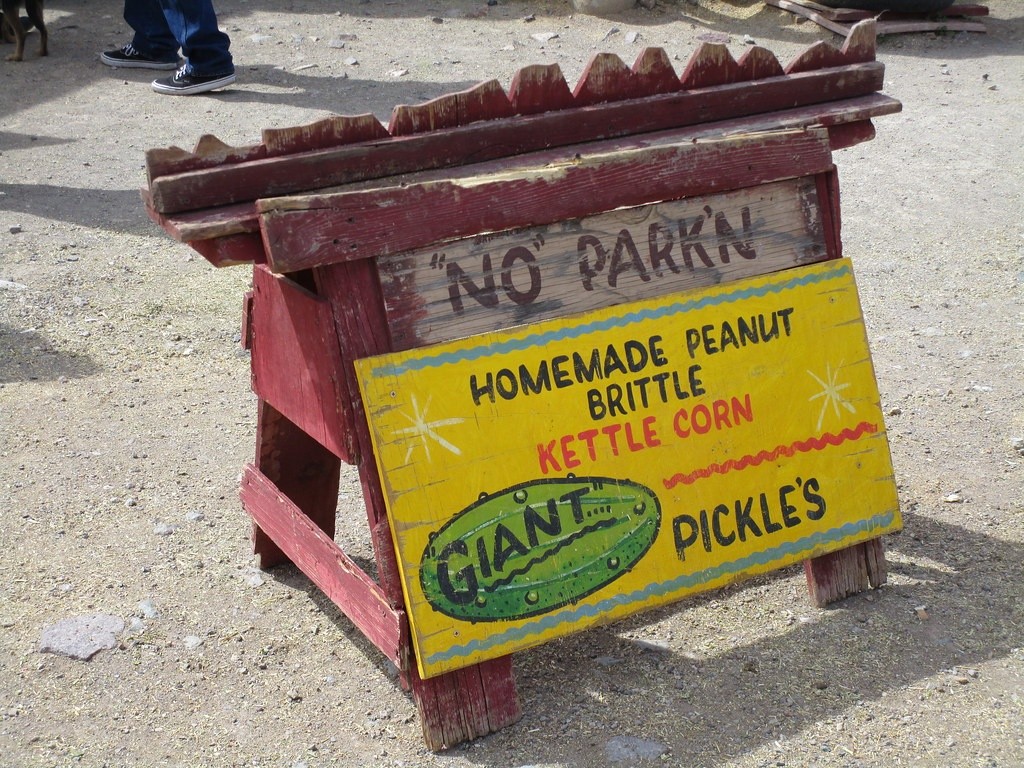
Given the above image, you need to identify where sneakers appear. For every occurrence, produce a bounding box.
[101,44,178,69]
[151,64,235,95]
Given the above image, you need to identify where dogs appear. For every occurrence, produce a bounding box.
[0,0,48,61]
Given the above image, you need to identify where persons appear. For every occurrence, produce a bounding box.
[100,0,235,95]
